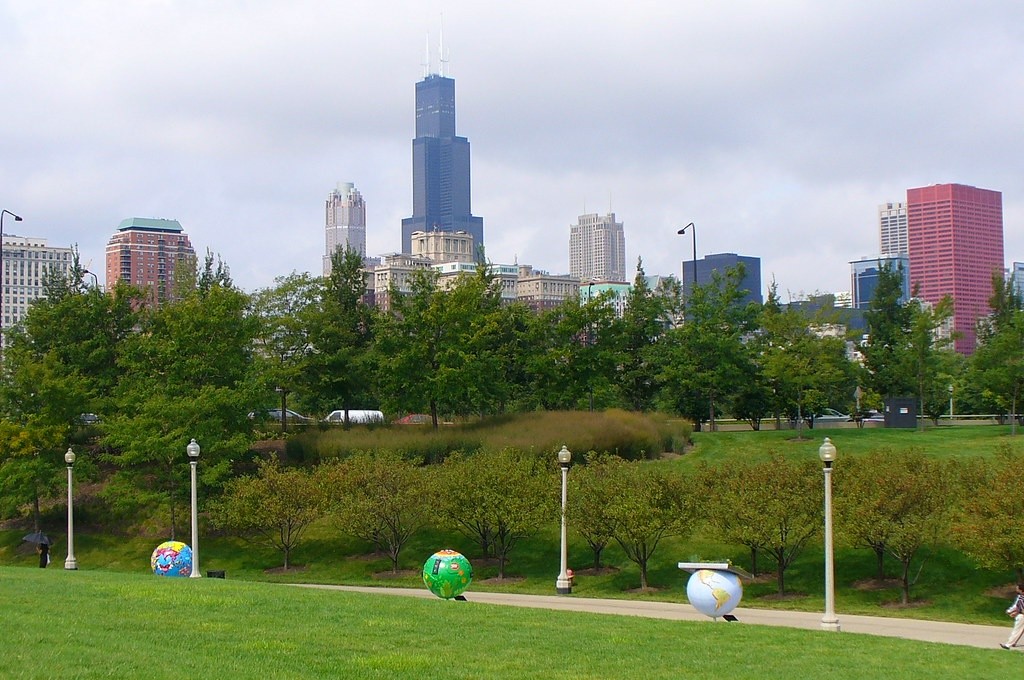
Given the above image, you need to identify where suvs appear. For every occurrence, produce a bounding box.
[245,407,313,425]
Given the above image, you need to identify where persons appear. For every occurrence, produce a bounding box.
[999,583,1024,649]
[36,544,50,568]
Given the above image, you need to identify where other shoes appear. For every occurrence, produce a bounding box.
[1000,644,1009,650]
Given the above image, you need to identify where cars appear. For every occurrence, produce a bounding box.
[391,414,444,426]
[849,410,885,420]
[73,413,102,426]
[800,407,855,423]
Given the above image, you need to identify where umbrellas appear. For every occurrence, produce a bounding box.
[22,531,53,547]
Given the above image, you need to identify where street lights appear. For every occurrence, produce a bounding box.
[678,222,697,288]
[556,445,573,594]
[0,209,22,352]
[186,436,204,579]
[818,436,839,624]
[588,281,595,413]
[948,384,954,420]
[64,447,78,570]
[83,269,98,306]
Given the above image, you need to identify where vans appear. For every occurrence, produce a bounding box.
[320,409,385,426]
